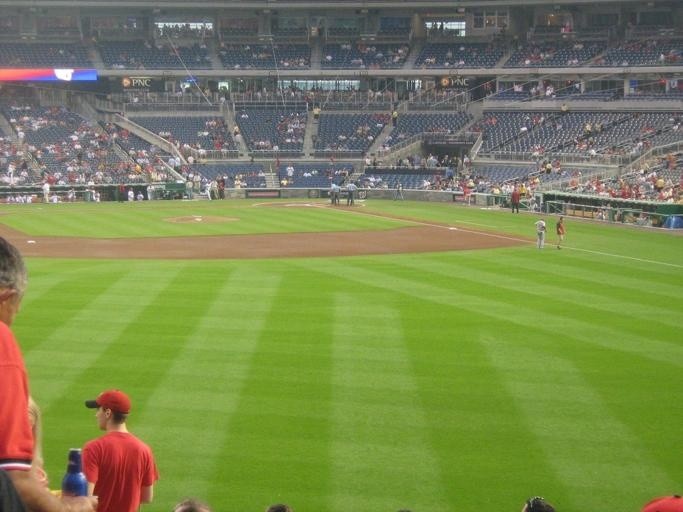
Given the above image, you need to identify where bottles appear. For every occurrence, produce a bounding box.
[62,449,88,496]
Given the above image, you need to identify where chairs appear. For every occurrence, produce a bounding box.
[0,27,682,203]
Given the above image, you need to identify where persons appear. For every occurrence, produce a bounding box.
[531,218,548,249]
[554,214,566,251]
[2,7,521,212]
[1,236,99,512]
[79,387,157,512]
[513,20,683,228]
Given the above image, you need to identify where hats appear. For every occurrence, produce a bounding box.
[84,388,133,414]
